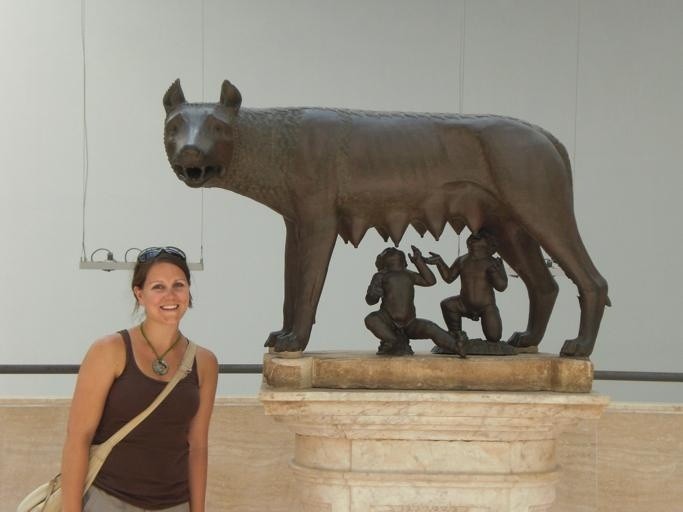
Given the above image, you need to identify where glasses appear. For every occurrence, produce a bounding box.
[139,246,186,262]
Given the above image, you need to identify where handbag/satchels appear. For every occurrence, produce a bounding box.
[16,444,111,512]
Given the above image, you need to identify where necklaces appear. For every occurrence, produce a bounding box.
[140,322,181,375]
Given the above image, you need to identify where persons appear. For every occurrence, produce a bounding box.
[422,232,507,343]
[365,245,467,359]
[62,245,219,512]
